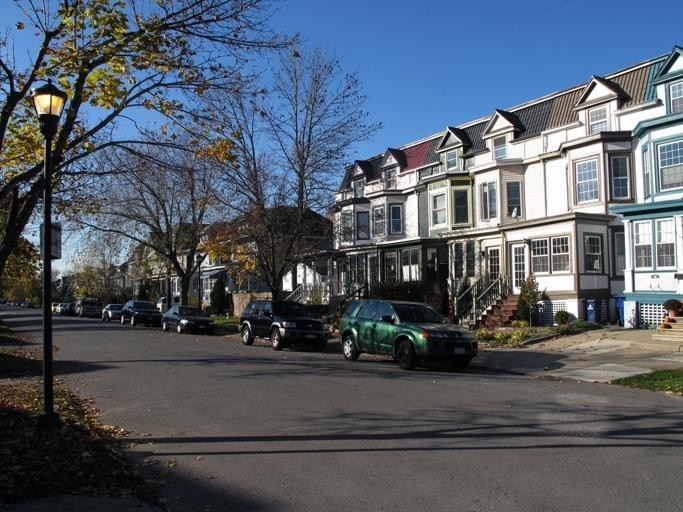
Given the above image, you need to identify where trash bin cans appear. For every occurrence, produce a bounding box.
[583,294,626,327]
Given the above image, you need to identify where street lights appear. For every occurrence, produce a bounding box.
[193,252,210,312]
[27,76,75,435]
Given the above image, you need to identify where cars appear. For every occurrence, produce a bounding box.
[158,304,218,338]
[100,302,125,323]
[2,300,34,309]
[51,296,104,319]
[120,299,163,328]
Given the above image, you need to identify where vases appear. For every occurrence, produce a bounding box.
[661,323,666,328]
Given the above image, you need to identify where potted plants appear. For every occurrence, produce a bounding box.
[663,299,683,317]
[664,313,670,323]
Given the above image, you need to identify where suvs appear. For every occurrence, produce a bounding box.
[336,297,484,375]
[239,295,329,354]
